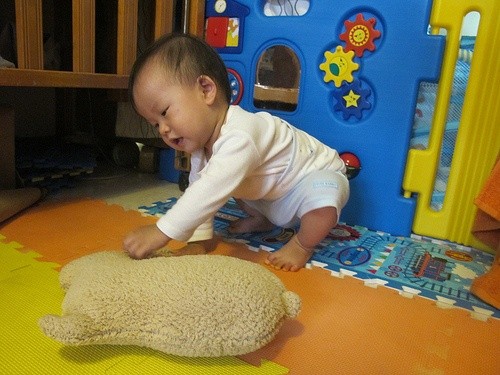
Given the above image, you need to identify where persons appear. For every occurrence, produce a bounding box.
[123,32,351,271]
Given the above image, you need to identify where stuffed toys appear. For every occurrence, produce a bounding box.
[41,250,302,357]
